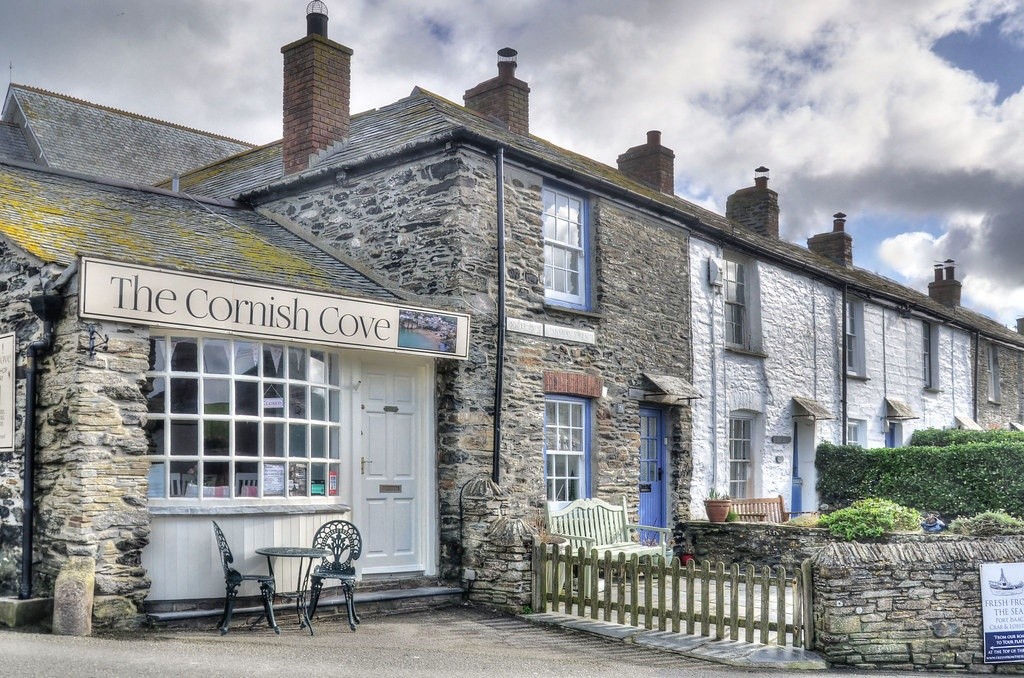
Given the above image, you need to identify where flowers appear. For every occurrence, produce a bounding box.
[186,463,216,487]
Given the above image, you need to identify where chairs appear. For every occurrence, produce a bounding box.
[169,472,181,495]
[235,472,258,497]
[299,519,363,633]
[211,520,281,635]
[181,473,218,496]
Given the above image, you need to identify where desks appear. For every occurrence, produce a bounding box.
[249,546,333,636]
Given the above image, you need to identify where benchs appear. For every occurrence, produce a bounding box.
[543,495,672,598]
[728,494,817,522]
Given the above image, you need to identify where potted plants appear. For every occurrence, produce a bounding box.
[526,515,571,594]
[681,538,695,564]
[703,487,733,523]
[642,518,676,567]
